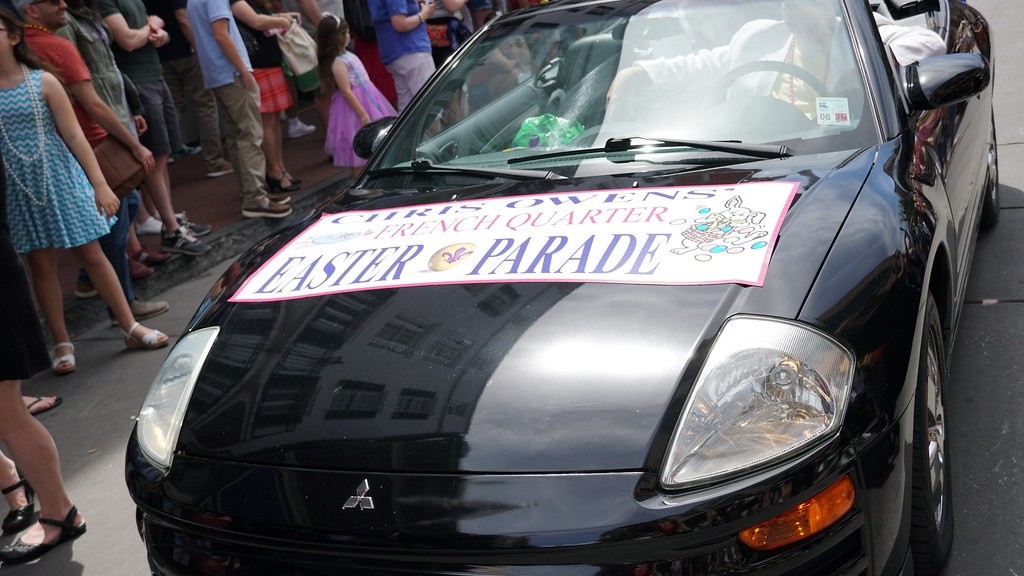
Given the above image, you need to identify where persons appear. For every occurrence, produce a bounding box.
[0,226,87,565]
[0,5,169,376]
[0,0,548,327]
[606,0,947,120]
[22,395,63,415]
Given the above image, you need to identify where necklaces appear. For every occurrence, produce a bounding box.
[790,40,829,104]
[0,64,45,161]
[24,24,47,31]
[2,63,47,206]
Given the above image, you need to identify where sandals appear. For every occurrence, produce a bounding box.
[1,463,34,533]
[0,504,86,564]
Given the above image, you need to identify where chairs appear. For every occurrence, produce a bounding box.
[548,31,625,146]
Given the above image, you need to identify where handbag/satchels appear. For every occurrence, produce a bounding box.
[93,134,145,201]
[272,14,322,92]
[510,114,585,148]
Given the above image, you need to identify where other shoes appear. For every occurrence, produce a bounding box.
[174,145,202,155]
[168,157,173,163]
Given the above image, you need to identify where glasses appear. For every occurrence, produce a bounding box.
[24,0,60,13]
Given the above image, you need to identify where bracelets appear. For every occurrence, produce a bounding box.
[417,13,423,24]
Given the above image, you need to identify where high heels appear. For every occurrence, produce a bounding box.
[52,342,76,374]
[120,322,169,350]
[266,175,299,192]
[284,169,302,183]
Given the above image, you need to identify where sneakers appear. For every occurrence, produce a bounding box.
[206,161,234,177]
[242,197,292,217]
[111,295,169,327]
[75,281,98,297]
[125,209,212,281]
[264,192,292,203]
[288,119,315,138]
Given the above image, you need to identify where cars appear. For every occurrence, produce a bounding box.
[124,0,997,576]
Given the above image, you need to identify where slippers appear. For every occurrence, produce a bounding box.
[27,396,64,416]
